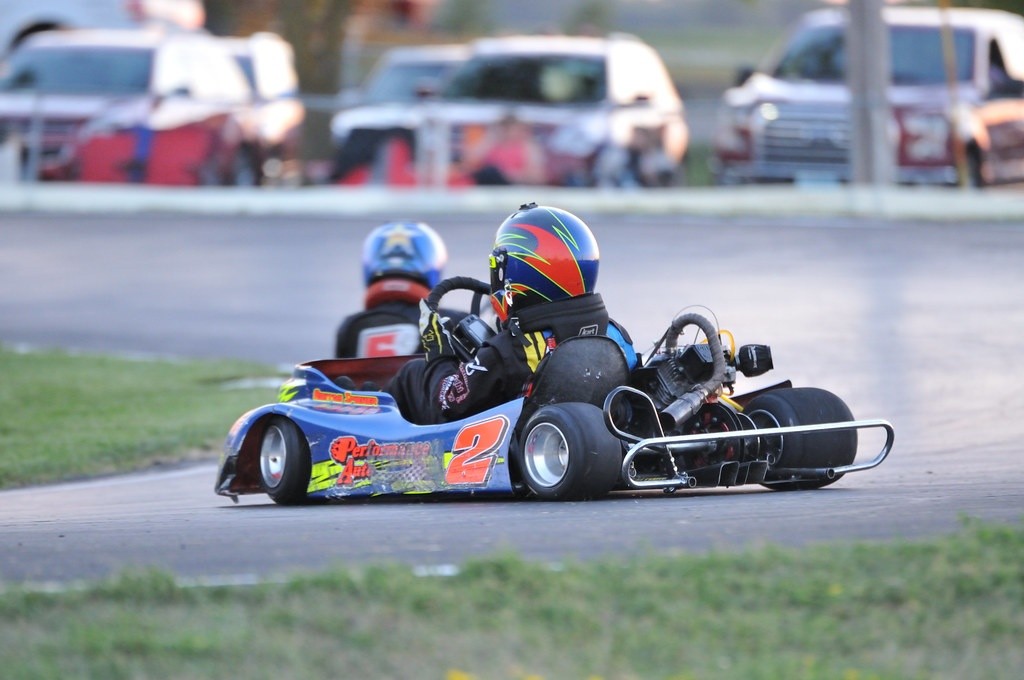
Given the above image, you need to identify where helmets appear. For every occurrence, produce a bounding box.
[359,220,447,290]
[489,202,600,325]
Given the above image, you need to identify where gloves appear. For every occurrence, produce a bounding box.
[419,298,456,364]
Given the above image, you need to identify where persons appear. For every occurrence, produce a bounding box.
[332,202,636,427]
[597,122,672,188]
[335,221,469,359]
[465,115,546,185]
[402,82,450,189]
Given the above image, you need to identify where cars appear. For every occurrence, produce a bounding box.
[0,0,690,187]
[708,4,1024,193]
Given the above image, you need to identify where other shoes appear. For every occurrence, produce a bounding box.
[361,382,381,392]
[334,377,357,393]
